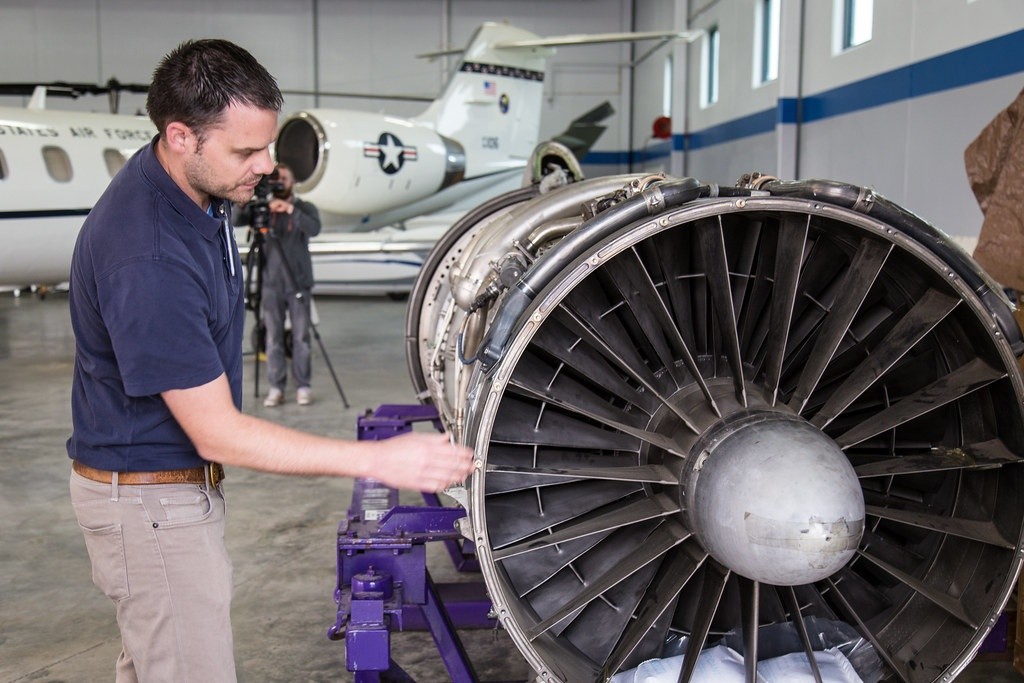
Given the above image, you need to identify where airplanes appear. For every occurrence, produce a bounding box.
[0,18,707,298]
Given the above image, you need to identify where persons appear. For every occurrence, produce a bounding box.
[66,37,477,683]
[963,86,1024,306]
[232,164,321,407]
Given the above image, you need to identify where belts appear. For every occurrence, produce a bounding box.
[72,459,225,488]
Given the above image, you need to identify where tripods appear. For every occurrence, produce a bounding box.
[243,201,350,410]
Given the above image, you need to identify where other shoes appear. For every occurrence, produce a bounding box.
[297,387,312,405]
[264,388,283,407]
